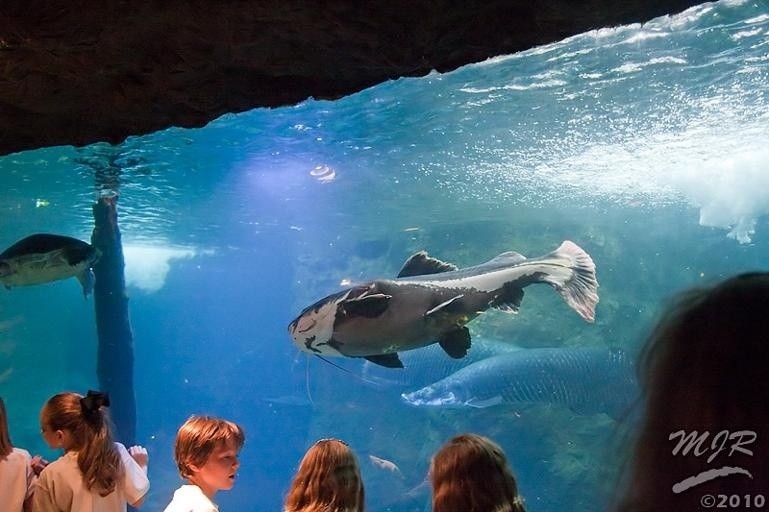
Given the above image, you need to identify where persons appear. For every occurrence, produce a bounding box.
[610,276,767,512]
[163,414,245,510]
[428,433,524,511]
[31,390,150,512]
[0,396,38,512]
[283,438,366,512]
